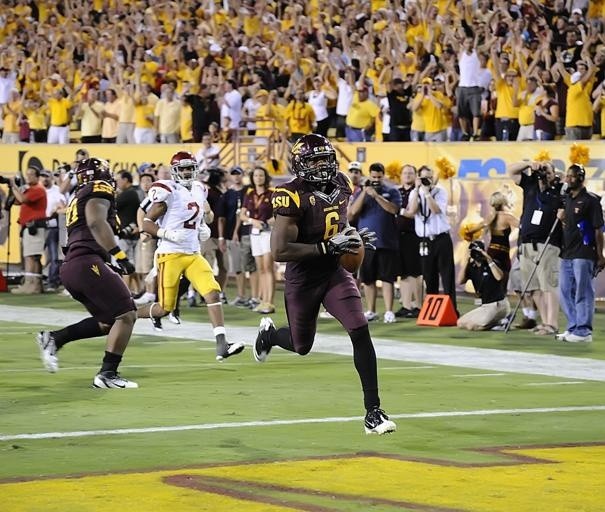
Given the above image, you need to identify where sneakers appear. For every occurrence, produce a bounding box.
[216,343,244,361]
[254,318,276,361]
[364,306,420,323]
[131,291,156,304]
[220,296,275,314]
[365,408,396,434]
[93,373,138,389]
[189,297,196,307]
[11,283,41,295]
[515,316,592,343]
[38,331,58,373]
[150,312,180,330]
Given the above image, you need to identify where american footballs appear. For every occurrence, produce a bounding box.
[339,230,364,275]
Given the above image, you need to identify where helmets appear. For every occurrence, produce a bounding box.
[76,158,113,184]
[289,134,339,182]
[170,152,197,186]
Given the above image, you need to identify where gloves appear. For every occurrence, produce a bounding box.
[317,227,377,255]
[199,224,211,241]
[157,229,185,242]
[110,246,135,275]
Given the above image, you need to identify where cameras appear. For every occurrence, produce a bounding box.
[372,181,382,195]
[469,239,485,262]
[0,176,20,186]
[120,222,137,237]
[420,177,433,185]
[534,166,547,180]
[58,164,71,172]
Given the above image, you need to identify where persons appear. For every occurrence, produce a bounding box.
[137,149,246,364]
[252,133,398,437]
[0,0,605,343]
[35,157,141,389]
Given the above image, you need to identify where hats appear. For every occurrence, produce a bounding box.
[348,162,361,170]
[139,163,155,171]
[209,21,433,99]
[231,167,242,174]
[39,170,51,177]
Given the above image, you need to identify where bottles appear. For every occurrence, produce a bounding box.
[503,130,508,141]
